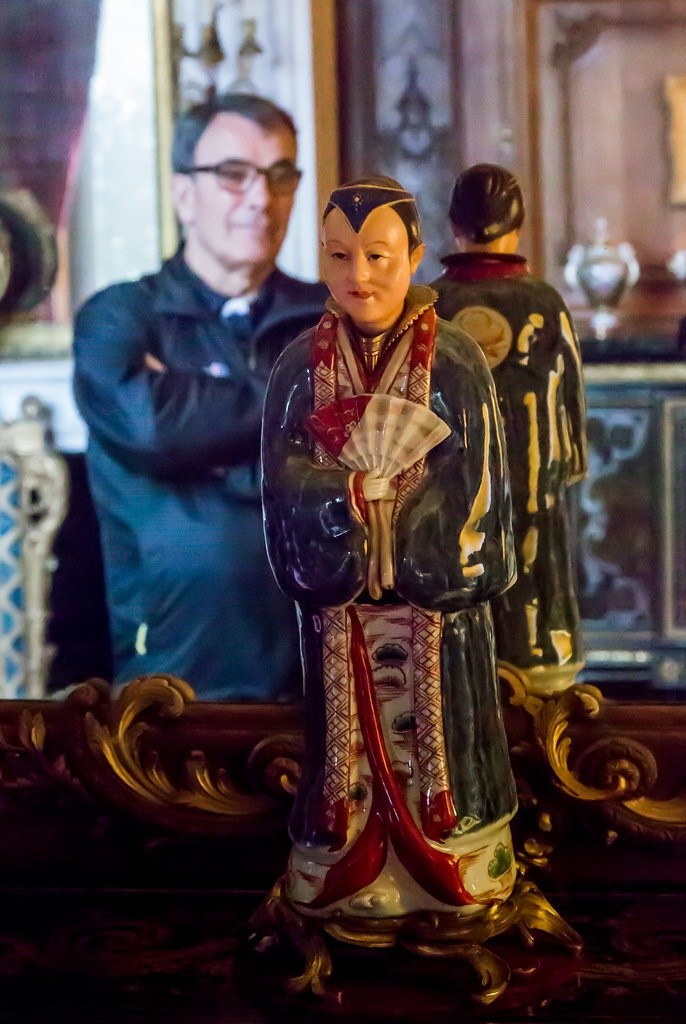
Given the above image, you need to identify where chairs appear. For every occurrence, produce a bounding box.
[0,395,70,698]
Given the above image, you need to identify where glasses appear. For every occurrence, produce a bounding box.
[181,161,301,195]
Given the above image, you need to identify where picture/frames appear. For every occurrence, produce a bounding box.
[152,0,344,285]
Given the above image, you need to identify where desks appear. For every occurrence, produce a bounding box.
[579,337,686,689]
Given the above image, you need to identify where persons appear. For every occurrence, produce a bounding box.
[426,164,660,805]
[72,93,319,702]
[248,172,580,1006]
